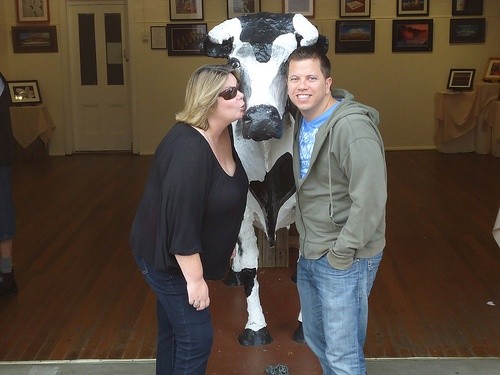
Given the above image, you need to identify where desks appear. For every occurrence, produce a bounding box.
[476,82,500,155]
[435,90,475,154]
[490,100,500,158]
[9,105,56,159]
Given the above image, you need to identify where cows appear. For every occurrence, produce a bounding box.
[199,13,329,346]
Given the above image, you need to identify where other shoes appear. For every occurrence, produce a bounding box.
[0,269,19,296]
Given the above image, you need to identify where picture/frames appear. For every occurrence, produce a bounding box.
[447,69,475,90]
[396,0,429,17]
[169,0,204,21]
[282,0,316,19]
[452,0,483,16]
[392,19,433,53]
[482,57,500,82]
[150,26,166,49]
[9,80,42,106]
[16,0,49,24]
[227,0,261,20]
[450,18,485,43]
[166,23,208,55]
[335,20,375,53]
[339,0,371,18]
[11,26,58,54]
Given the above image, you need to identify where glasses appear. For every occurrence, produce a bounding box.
[217,80,241,100]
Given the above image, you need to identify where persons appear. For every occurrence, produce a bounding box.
[0,72,19,301]
[283,43,388,375]
[128,63,249,375]
